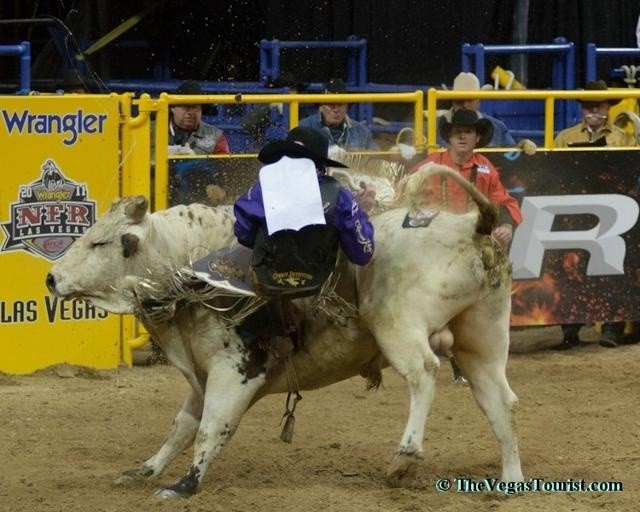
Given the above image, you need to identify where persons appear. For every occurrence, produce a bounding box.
[234,126,373,301]
[547,80,639,350]
[166,82,230,154]
[405,111,523,386]
[297,78,416,160]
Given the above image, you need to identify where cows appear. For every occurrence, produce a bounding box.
[44,159,527,503]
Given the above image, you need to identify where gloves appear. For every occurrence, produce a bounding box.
[517,137,537,157]
[387,143,417,161]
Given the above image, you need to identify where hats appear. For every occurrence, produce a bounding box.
[436,108,494,149]
[172,81,220,118]
[574,80,624,107]
[441,72,494,94]
[257,125,350,170]
[322,75,348,95]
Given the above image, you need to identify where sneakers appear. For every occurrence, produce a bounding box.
[553,321,627,351]
